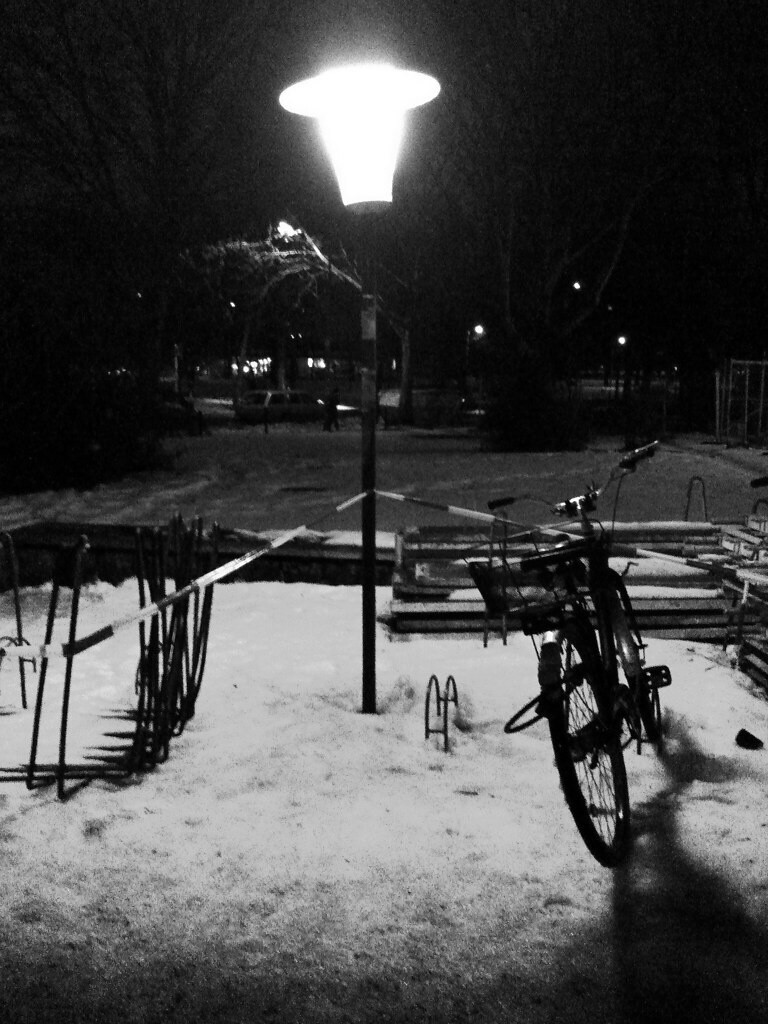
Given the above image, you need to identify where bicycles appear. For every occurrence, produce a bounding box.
[462,436,671,867]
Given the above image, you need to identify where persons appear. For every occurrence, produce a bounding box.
[323,388,340,432]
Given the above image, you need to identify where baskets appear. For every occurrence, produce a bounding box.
[459,518,609,632]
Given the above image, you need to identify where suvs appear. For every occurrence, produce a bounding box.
[239,390,316,405]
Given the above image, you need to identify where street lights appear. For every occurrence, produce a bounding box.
[278,62,450,713]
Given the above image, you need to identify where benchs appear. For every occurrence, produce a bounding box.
[392,523,731,639]
